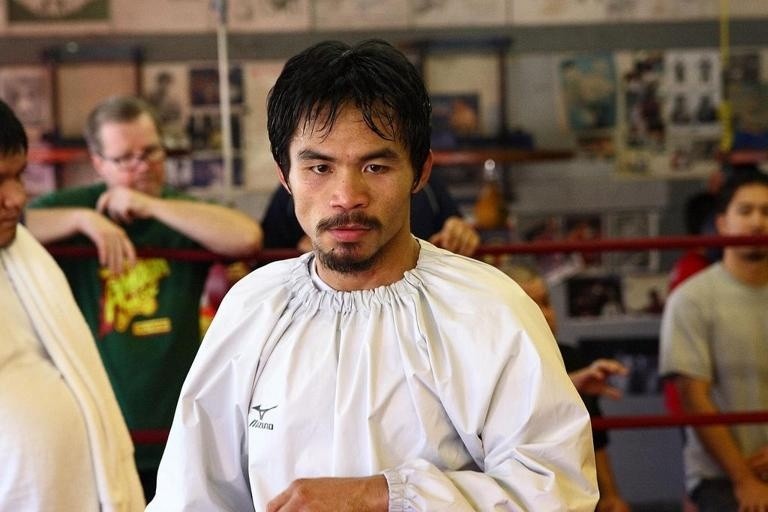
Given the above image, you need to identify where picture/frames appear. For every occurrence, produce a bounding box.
[0,42,62,197]
[390,44,508,197]
[136,62,248,194]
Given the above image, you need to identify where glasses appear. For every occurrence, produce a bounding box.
[98,147,165,169]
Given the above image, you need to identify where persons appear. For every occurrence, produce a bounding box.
[17,93,267,512]
[494,262,634,511]
[148,73,186,139]
[139,36,601,512]
[657,171,768,512]
[1,94,149,512]
[661,190,726,511]
[260,168,486,262]
